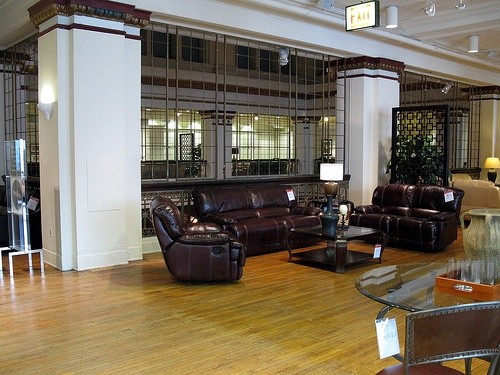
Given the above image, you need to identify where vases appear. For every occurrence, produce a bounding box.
[320,195,339,236]
[460,208,500,286]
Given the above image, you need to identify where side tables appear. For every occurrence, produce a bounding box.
[307,199,354,216]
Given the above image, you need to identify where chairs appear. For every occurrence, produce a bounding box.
[376,301,500,375]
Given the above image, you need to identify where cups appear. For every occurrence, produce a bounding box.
[445,256,500,285]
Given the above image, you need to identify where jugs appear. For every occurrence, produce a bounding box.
[460,208,500,285]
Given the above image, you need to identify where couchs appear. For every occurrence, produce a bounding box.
[150,196,246,281]
[350,184,465,253]
[448,169,500,221]
[192,185,323,256]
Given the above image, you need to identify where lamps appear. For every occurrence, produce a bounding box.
[37,103,52,121]
[320,163,343,202]
[442,84,452,94]
[339,205,347,237]
[423,0,435,18]
[456,0,465,10]
[278,48,289,66]
[468,35,478,53]
[483,157,500,183]
[386,5,398,29]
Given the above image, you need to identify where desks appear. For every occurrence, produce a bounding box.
[354,260,500,375]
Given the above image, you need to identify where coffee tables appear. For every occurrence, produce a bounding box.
[287,226,387,273]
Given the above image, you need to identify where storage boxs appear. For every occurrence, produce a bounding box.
[436,268,500,301]
